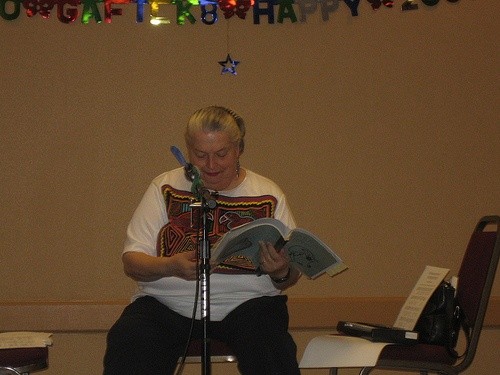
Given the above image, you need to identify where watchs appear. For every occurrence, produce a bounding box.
[270,265,291,283]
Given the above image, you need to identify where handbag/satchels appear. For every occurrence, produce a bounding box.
[415,281,470,361]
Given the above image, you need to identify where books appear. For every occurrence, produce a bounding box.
[210,218,348,283]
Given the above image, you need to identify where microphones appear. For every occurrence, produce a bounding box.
[184,165,217,209]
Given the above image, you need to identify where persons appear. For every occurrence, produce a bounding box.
[102,105,300,375]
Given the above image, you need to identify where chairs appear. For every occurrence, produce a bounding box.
[0,332,54,375]
[329,215,500,375]
